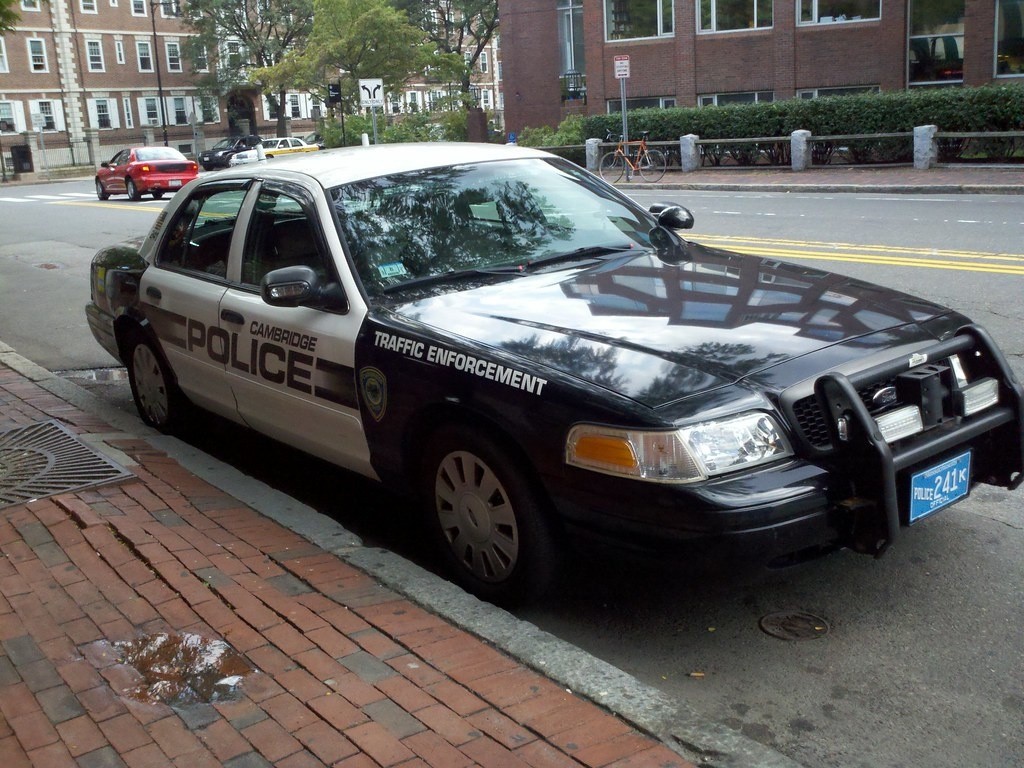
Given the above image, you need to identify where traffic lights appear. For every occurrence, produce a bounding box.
[328,83,339,92]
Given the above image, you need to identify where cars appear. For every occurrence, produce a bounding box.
[93,146,199,201]
[229,136,320,168]
[303,130,331,150]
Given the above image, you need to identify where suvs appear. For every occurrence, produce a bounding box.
[83,141,1024,614]
[198,135,263,171]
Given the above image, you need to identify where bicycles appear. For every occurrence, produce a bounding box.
[598,127,669,185]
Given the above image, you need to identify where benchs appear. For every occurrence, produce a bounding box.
[187,217,309,273]
